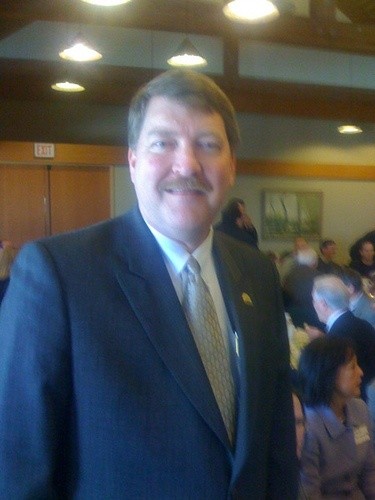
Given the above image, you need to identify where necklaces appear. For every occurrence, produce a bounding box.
[339,415,346,424]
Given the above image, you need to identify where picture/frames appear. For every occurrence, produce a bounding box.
[259,188,324,241]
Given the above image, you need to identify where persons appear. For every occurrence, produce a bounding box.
[0,196,375,334]
[295,334,375,500]
[0,71,304,500]
[311,276,375,394]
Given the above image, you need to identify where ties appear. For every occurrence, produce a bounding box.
[184,257,241,445]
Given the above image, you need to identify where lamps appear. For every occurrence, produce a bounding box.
[50,79,86,93]
[166,2,207,67]
[336,53,363,134]
[58,33,104,62]
[221,0,280,24]
